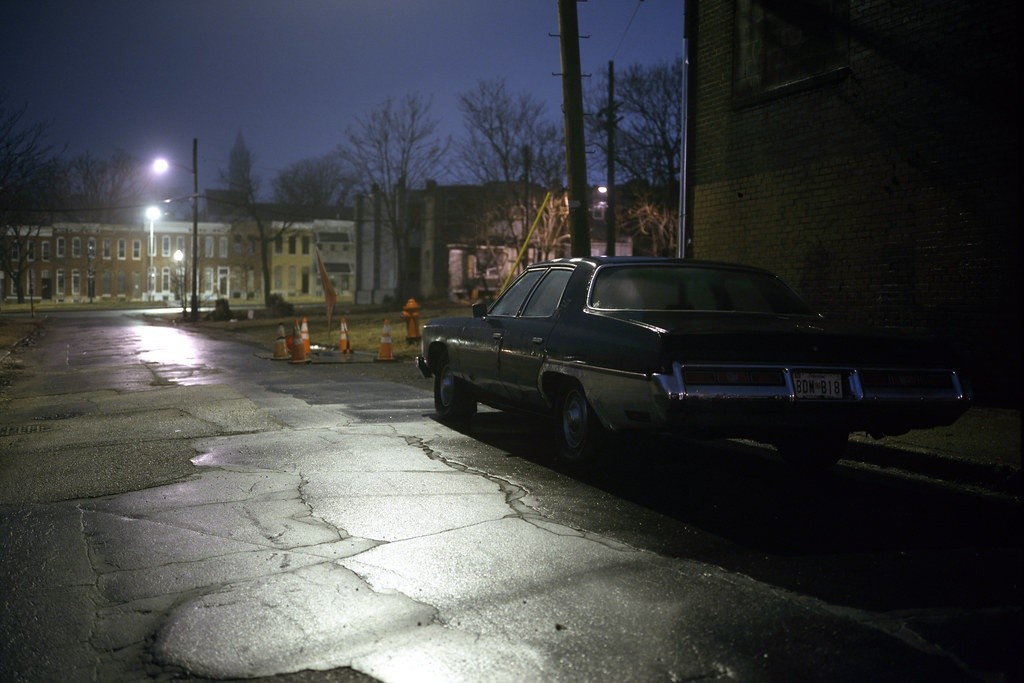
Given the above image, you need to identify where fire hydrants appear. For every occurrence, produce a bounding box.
[401,297,424,345]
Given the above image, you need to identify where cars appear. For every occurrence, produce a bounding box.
[416,256,977,468]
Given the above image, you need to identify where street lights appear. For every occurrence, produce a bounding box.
[150,155,199,322]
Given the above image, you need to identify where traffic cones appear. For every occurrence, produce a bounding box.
[288,319,312,364]
[271,323,290,359]
[374,318,397,364]
[298,316,312,356]
[338,317,354,355]
